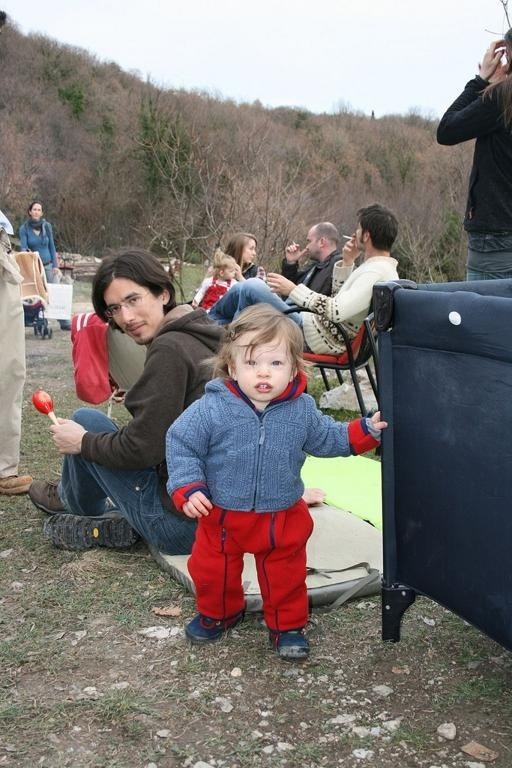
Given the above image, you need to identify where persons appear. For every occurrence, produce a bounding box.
[1,210,35,497]
[434,27,511,282]
[190,202,402,354]
[16,202,75,330]
[27,245,227,558]
[165,302,390,662]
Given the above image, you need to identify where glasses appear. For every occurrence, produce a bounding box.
[104,294,142,317]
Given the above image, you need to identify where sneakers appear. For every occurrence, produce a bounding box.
[186,611,243,641]
[270,630,310,658]
[1,475,139,551]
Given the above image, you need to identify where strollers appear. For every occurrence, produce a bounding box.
[12,251,53,339]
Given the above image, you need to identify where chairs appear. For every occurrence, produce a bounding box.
[282,307,403,441]
